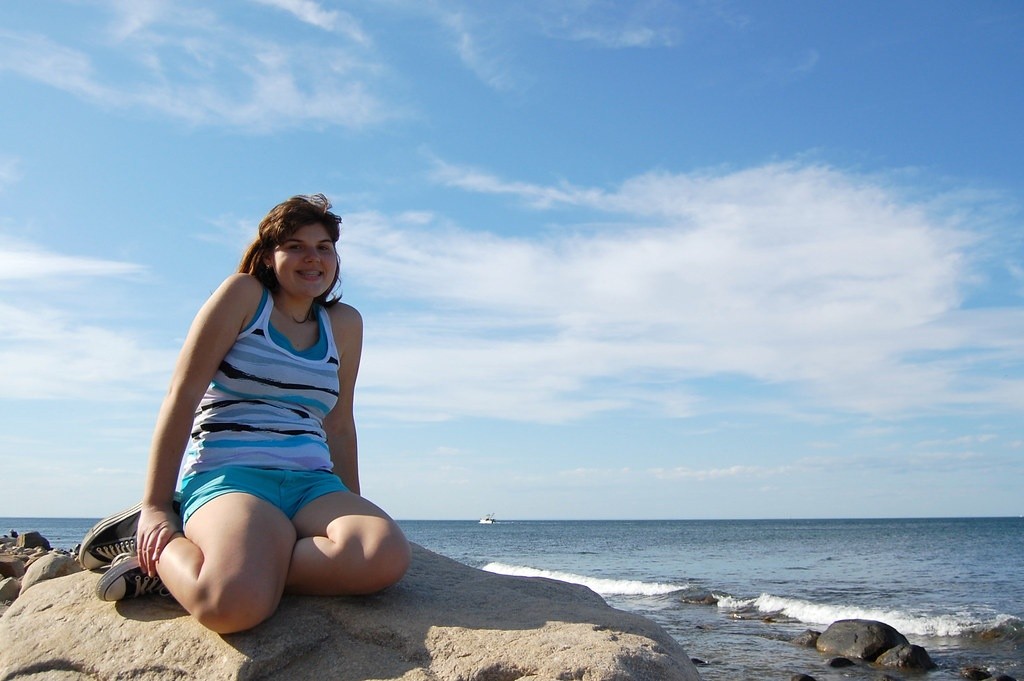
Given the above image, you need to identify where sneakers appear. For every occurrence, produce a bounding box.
[95,556,173,602]
[79,491,181,571]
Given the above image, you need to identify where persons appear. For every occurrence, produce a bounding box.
[9,530,18,538]
[80,193,412,633]
